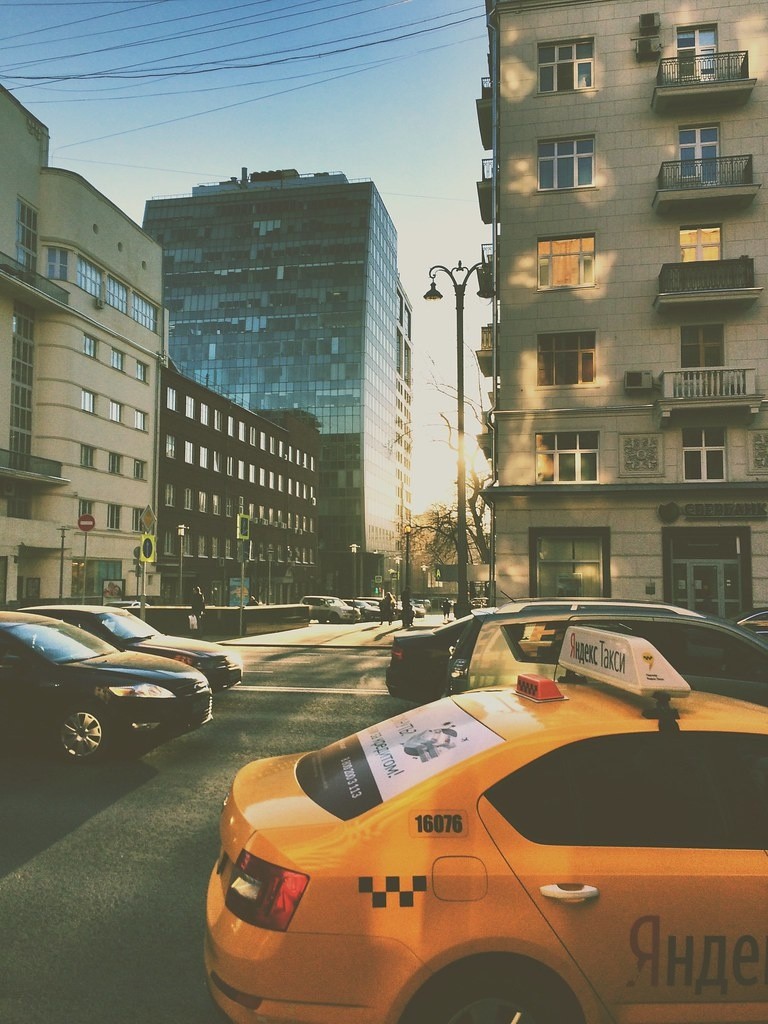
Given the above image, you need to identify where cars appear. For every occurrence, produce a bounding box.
[385,613,474,703]
[104,601,149,610]
[728,606,768,638]
[439,607,768,707]
[342,597,432,621]
[205,626,768,1024]
[15,604,244,693]
[0,611,214,764]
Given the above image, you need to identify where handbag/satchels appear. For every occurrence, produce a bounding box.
[189,614,197,630]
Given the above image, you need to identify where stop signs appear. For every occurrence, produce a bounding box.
[78,514,95,533]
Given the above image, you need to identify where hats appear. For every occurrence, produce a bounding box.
[193,587,200,592]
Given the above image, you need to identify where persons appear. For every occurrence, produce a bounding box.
[104,583,122,601]
[400,585,413,627]
[442,597,453,619]
[189,586,205,640]
[246,596,259,606]
[378,593,397,625]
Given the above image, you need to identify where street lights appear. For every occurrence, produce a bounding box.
[420,564,429,606]
[393,556,402,601]
[423,260,497,621]
[349,544,360,624]
[404,523,412,598]
[176,522,189,605]
[57,525,70,603]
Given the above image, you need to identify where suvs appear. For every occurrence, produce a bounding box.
[300,596,360,624]
[498,597,707,620]
